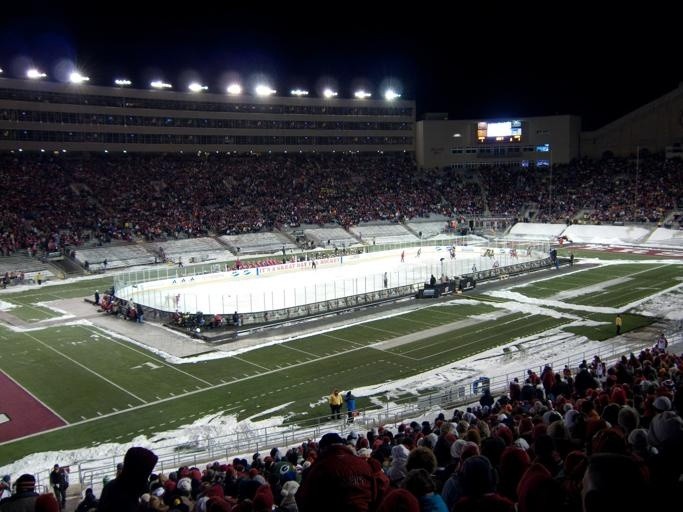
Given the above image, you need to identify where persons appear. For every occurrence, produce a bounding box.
[1,152,682,511]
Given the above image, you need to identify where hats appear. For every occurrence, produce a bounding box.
[164,452,273,511]
